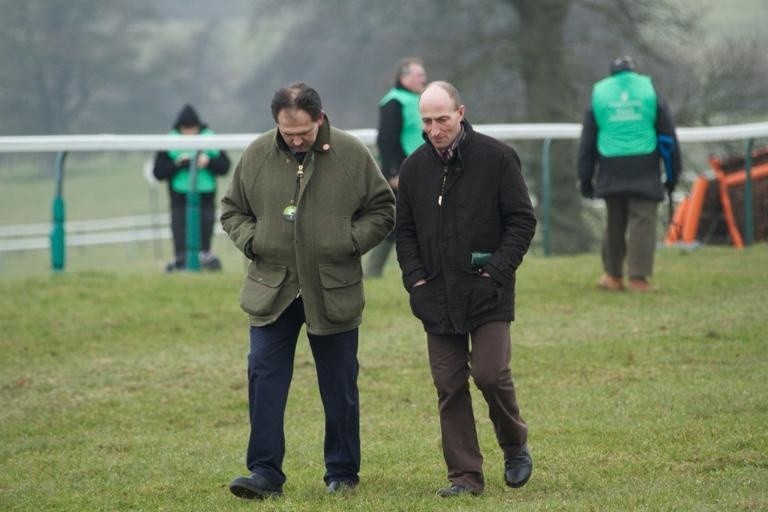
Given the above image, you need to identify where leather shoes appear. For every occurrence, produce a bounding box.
[229,471,283,498]
[325,480,354,495]
[504,447,532,489]
[436,484,474,498]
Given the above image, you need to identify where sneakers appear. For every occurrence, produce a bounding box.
[628,277,659,294]
[597,272,624,292]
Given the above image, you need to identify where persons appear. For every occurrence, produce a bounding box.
[396,77,537,499]
[217,81,397,502]
[365,58,432,280]
[151,105,231,270]
[574,54,682,291]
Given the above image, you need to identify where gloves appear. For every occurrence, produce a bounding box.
[664,181,676,193]
[579,180,595,199]
